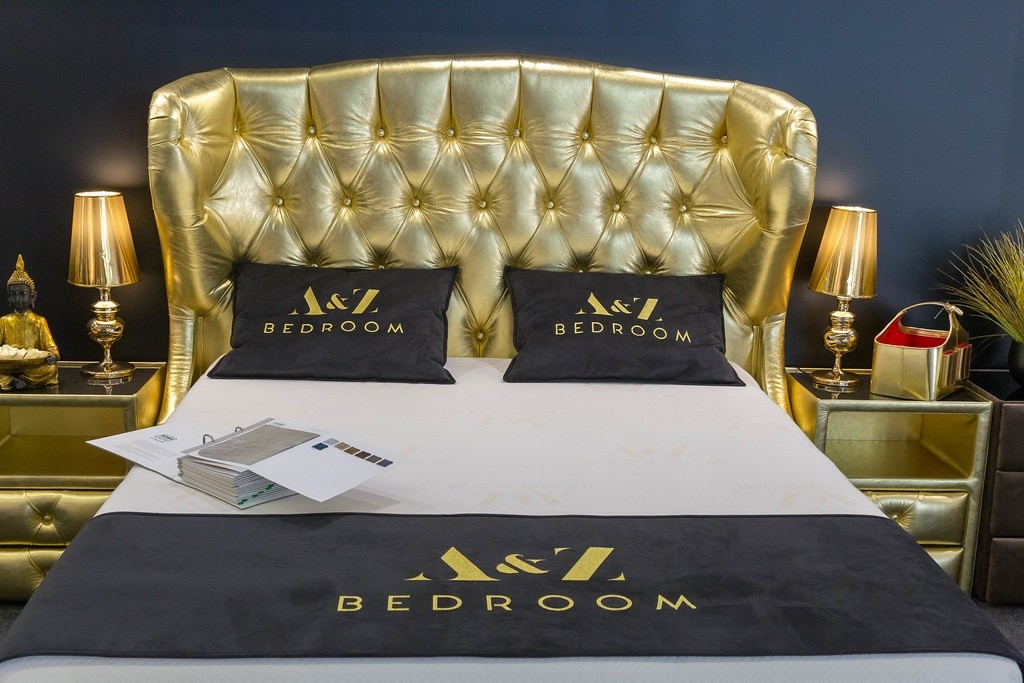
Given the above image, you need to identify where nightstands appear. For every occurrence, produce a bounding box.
[0,368,166,603]
[785,372,995,594]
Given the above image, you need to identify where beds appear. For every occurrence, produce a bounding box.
[0,55,1024,683]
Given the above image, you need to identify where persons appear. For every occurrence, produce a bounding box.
[0,271,59,390]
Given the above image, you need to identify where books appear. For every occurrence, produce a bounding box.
[85,397,393,510]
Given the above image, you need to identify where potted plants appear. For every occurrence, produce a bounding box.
[928,218,1024,388]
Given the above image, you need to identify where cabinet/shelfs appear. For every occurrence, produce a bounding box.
[965,370,1024,607]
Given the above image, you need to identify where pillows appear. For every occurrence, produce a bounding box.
[501,269,748,389]
[208,264,461,384]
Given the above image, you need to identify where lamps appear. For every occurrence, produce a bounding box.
[807,203,880,386]
[65,189,143,376]
